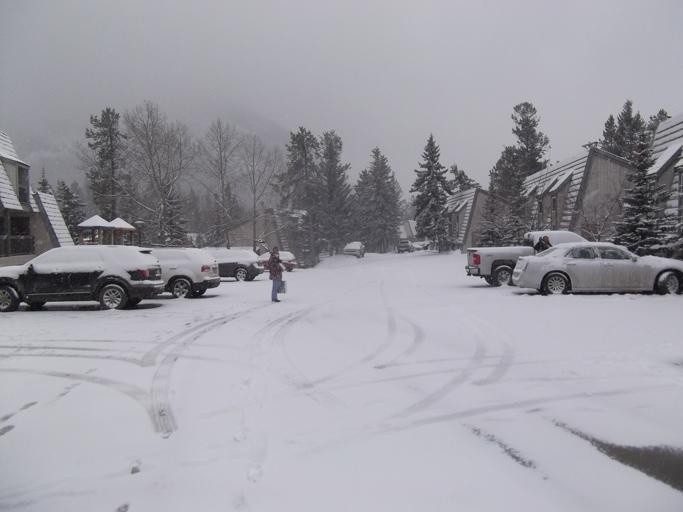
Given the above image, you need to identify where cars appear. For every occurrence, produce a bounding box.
[342,241,365,258]
[513,235,682,301]
[396,239,413,253]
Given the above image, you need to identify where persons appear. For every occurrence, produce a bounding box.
[534,235,552,253]
[267,245,285,304]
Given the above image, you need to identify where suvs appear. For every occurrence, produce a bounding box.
[1,244,295,311]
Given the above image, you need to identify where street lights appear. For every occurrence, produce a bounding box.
[133,220,145,246]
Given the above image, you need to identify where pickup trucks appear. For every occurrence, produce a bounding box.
[462,230,592,289]
